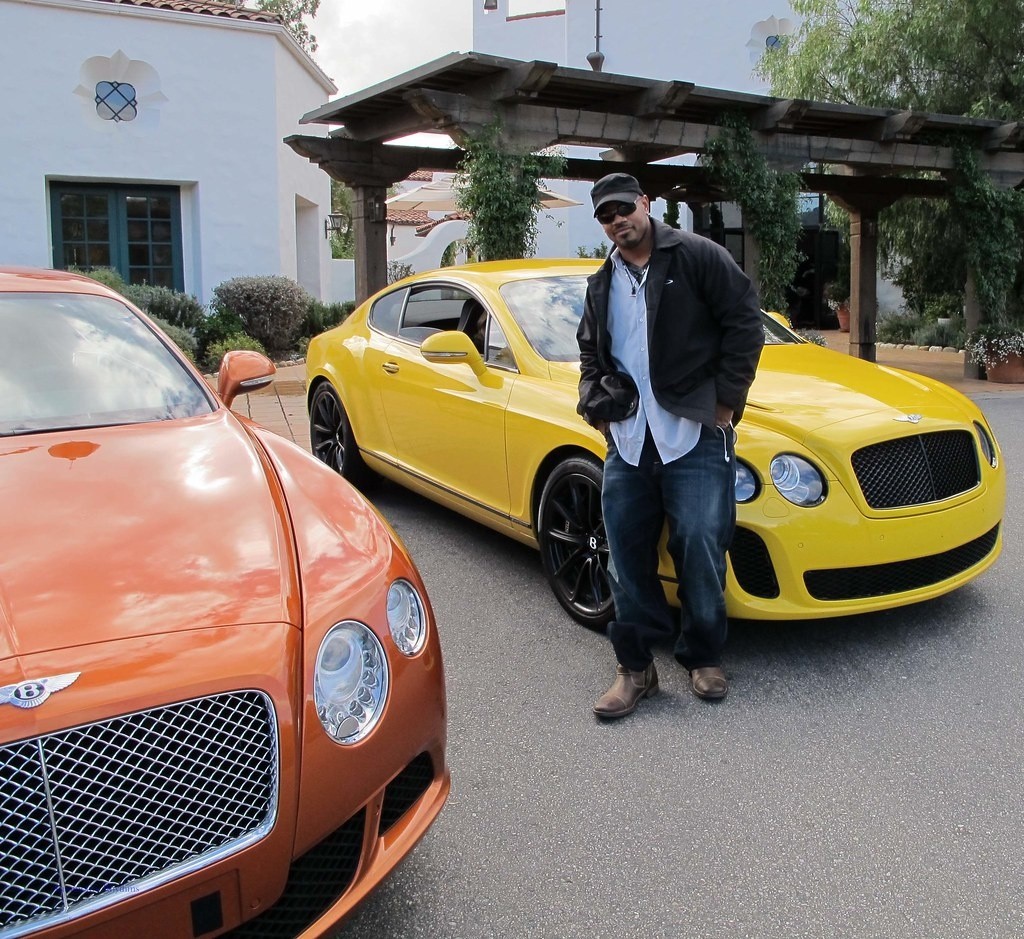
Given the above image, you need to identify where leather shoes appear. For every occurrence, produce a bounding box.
[592,661,660,718]
[689,666,729,700]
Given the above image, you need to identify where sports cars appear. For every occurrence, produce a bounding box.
[0,266,454,939]
[306,257,1007,632]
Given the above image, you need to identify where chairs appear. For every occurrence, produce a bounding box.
[458,298,486,353]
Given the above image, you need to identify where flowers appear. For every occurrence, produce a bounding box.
[824,280,880,312]
[965,323,1024,373]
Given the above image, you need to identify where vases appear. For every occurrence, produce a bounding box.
[984,351,1024,383]
[836,304,850,331]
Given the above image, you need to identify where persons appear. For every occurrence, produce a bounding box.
[577,172,765,717]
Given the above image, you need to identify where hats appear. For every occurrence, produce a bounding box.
[589,172,645,218]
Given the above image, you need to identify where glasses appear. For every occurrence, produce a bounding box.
[596,197,642,224]
